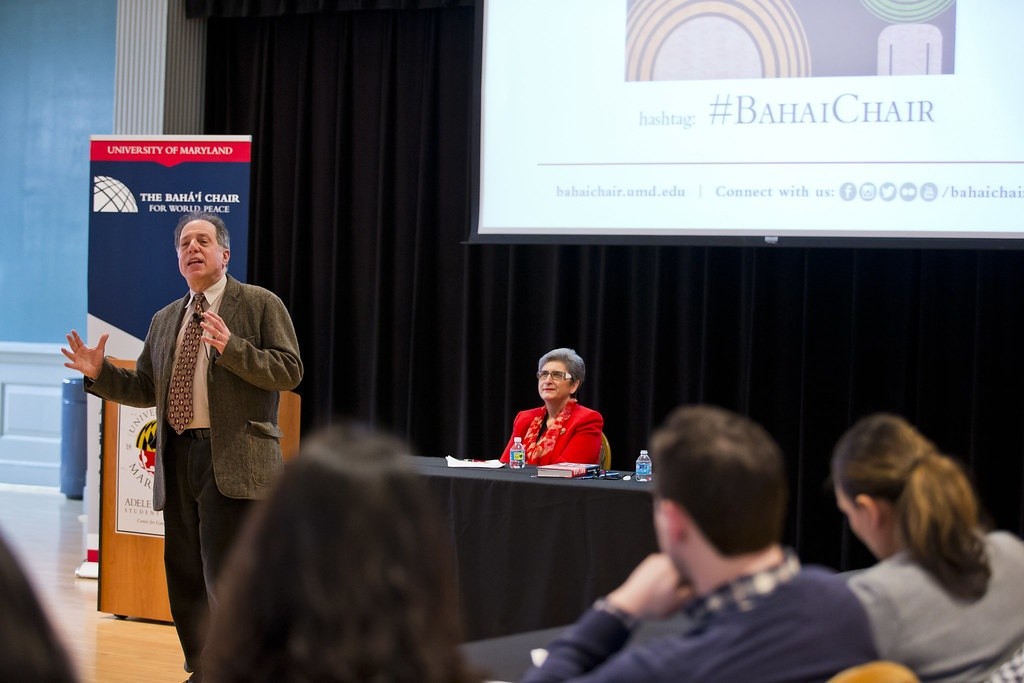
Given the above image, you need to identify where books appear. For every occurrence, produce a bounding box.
[537,463,601,478]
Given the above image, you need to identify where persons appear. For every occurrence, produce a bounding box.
[197,424,477,683]
[61,212,304,683]
[521,406,878,683]
[500,348,604,466]
[831,413,1024,683]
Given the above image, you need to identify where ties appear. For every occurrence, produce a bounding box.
[167,293,205,435]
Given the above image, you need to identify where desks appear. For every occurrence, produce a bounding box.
[410,456,660,645]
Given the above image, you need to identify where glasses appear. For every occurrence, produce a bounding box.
[535,369,573,381]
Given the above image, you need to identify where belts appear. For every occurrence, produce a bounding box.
[173,428,211,441]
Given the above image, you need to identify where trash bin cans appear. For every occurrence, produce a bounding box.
[60,377,87,501]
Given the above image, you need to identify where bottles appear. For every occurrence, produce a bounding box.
[635,450,652,482]
[510,437,525,469]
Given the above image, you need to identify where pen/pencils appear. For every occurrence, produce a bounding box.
[468,459,491,462]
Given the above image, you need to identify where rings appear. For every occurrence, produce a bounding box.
[216,332,220,337]
